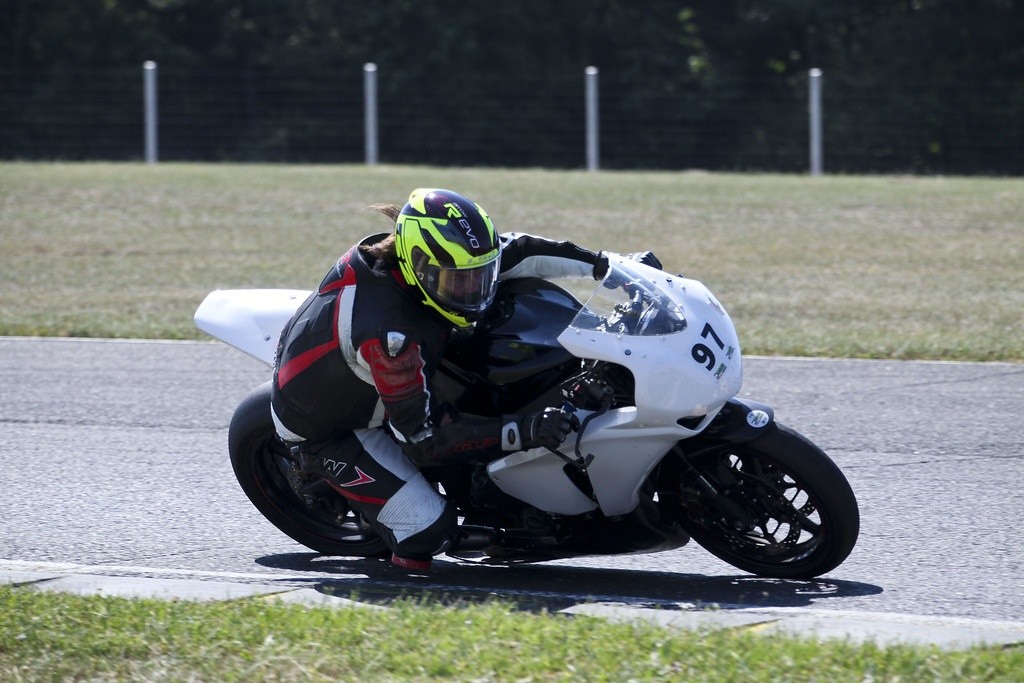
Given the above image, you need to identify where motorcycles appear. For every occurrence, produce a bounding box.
[194,251,860,581]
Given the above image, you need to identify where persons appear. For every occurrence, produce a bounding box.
[269,188,664,582]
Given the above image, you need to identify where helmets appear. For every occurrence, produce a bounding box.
[395,188,502,328]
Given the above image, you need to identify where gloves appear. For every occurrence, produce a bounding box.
[501,407,581,451]
[593,249,662,289]
